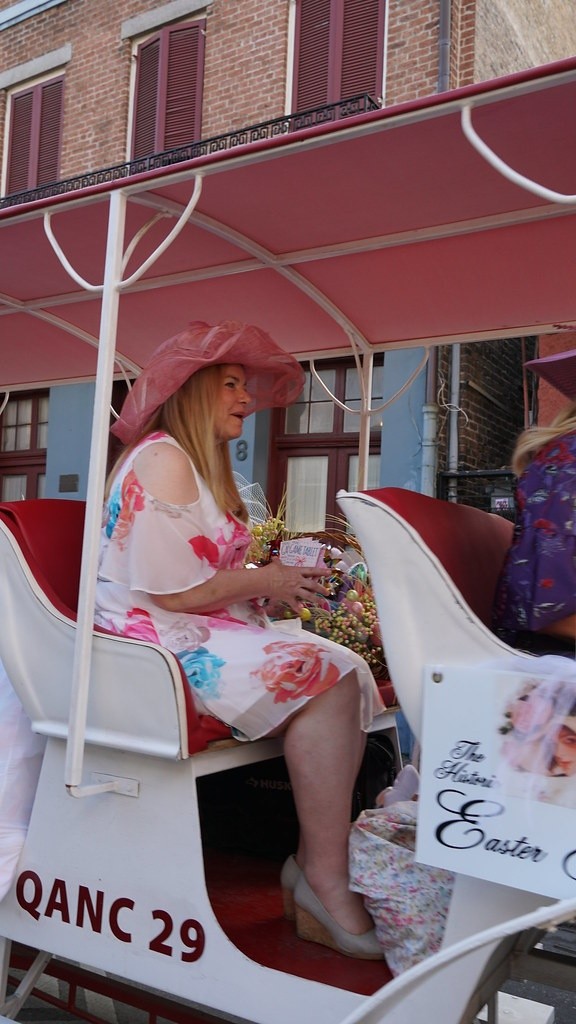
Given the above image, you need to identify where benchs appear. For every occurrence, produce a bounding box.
[0,498,398,762]
[335,486,576,737]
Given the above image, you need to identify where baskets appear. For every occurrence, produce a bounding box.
[260,511,390,681]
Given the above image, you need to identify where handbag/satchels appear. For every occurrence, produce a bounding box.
[348,801,456,979]
[219,731,397,864]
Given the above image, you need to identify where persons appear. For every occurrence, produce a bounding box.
[96,323,384,959]
[494,351,576,659]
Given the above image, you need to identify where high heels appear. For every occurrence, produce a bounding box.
[293,870,385,959]
[281,854,302,921]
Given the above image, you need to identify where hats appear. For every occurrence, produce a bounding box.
[110,320,306,445]
[523,349,576,403]
[516,713,576,776]
